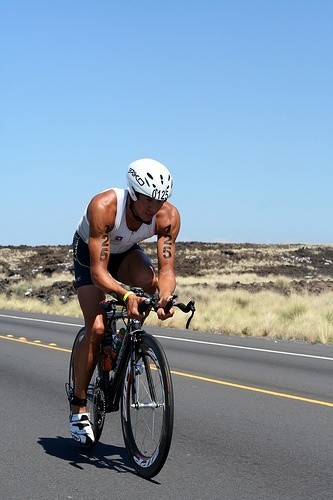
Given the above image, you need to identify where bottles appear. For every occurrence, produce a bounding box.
[101,338,113,372]
[111,328,126,361]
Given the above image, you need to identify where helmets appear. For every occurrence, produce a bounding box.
[126,158,174,202]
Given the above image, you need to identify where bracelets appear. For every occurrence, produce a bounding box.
[122,291,134,305]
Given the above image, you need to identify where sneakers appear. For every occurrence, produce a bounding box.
[68,410,96,446]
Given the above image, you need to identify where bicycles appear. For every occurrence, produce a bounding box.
[64,286,196,479]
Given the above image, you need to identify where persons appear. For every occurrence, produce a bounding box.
[68,158,181,445]
